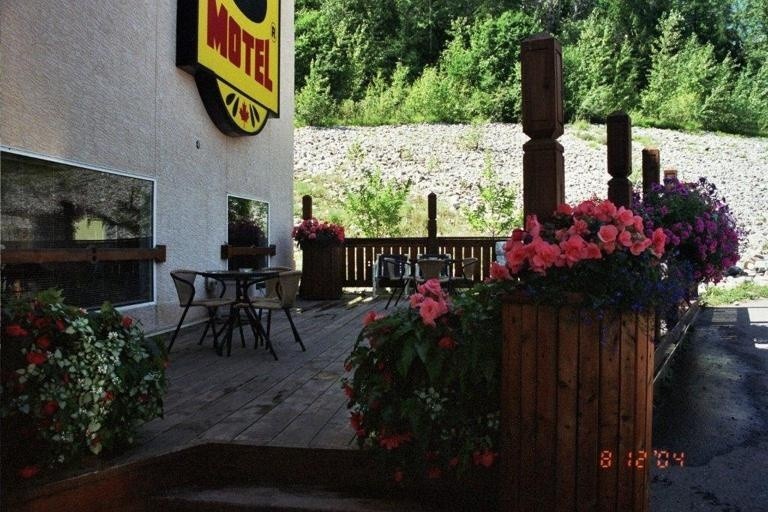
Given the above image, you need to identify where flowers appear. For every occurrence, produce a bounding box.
[291,218,346,251]
[343,178,748,506]
[0,280,169,485]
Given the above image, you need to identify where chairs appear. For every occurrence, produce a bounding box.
[375,252,481,309]
[161,266,310,364]
